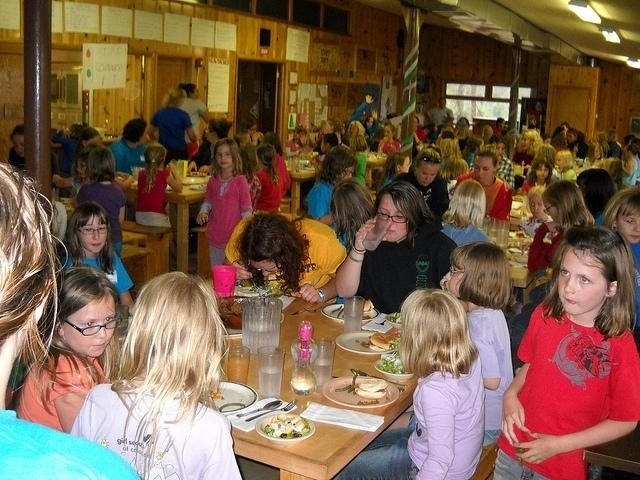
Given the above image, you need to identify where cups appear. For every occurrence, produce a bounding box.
[241,298,282,355]
[211,264,236,295]
[258,345,286,398]
[315,336,336,388]
[225,344,252,386]
[342,296,363,333]
[480,217,522,255]
[115,305,130,346]
[364,215,392,251]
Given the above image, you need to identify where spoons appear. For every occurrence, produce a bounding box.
[238,399,284,418]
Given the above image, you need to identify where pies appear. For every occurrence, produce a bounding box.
[363,299,377,319]
[354,380,388,399]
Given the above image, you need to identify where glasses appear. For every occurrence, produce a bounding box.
[77,224,109,234]
[543,206,553,215]
[64,313,123,336]
[376,212,408,223]
[449,267,463,276]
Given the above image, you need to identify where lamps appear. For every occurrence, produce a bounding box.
[625,56,639,71]
[568,0,601,25]
[597,25,621,45]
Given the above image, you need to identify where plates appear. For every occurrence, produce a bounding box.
[322,376,400,408]
[166,171,208,193]
[223,310,284,339]
[323,304,416,382]
[212,380,259,415]
[255,414,314,445]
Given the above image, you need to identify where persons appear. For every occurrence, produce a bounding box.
[2,83,640,447]
[333,287,485,480]
[68,271,242,480]
[0,163,142,480]
[492,224,640,480]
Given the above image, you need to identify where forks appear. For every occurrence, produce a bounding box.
[245,401,297,422]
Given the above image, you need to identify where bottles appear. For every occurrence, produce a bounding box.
[290,320,317,397]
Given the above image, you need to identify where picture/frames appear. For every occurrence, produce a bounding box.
[354,44,379,76]
[548,84,592,137]
